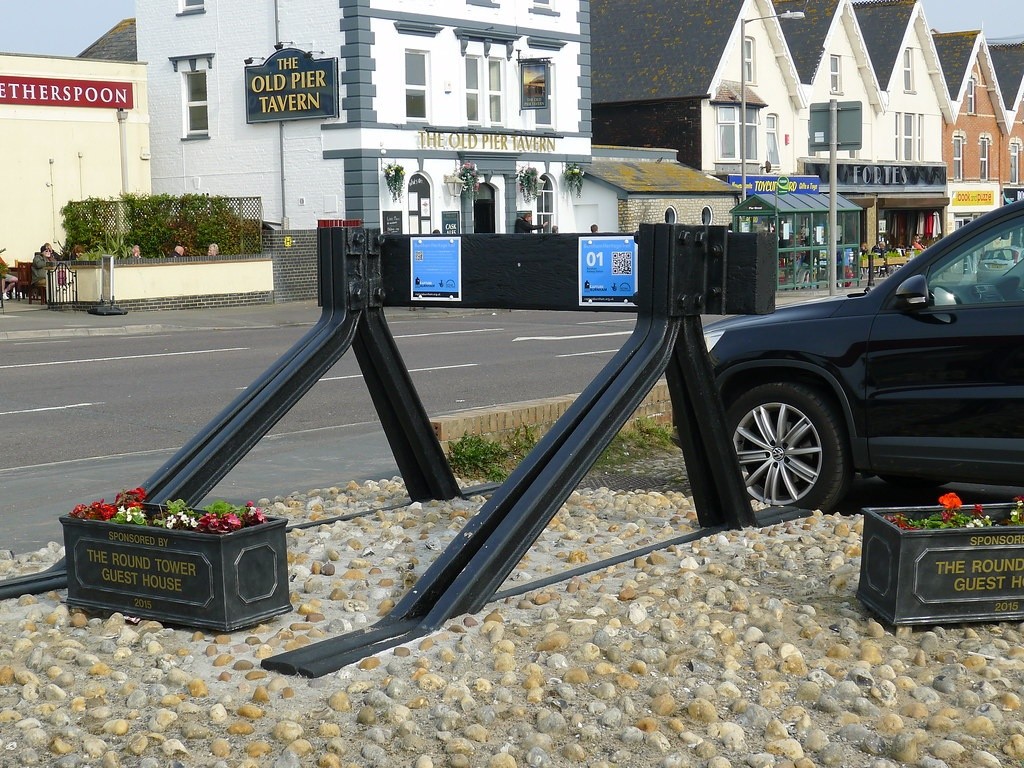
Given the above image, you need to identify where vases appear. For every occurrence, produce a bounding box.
[58,502,293,632]
[855,502,1024,625]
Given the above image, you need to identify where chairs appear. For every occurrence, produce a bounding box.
[10,262,28,301]
[27,261,45,305]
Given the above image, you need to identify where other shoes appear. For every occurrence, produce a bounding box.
[2,293,9,300]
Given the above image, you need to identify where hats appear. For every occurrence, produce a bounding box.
[41,246,47,253]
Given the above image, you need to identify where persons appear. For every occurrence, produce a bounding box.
[514,213,549,233]
[779,231,800,262]
[30,242,61,287]
[128,245,142,260]
[208,244,219,257]
[552,225,558,233]
[591,225,598,233]
[432,230,440,233]
[836,235,852,262]
[860,233,928,277]
[74,245,89,261]
[168,246,185,258]
[0,248,18,300]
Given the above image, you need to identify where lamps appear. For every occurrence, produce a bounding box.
[544,132,556,136]
[515,131,522,133]
[424,127,437,130]
[245,57,264,64]
[302,50,324,58]
[537,178,546,195]
[275,41,294,51]
[444,172,466,196]
[468,128,476,131]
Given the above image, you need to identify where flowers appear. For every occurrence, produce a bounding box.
[70,486,268,534]
[519,168,537,202]
[385,164,405,203]
[563,163,583,198]
[884,494,1024,529]
[458,163,479,202]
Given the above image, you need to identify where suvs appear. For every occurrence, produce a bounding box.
[662,198,1024,511]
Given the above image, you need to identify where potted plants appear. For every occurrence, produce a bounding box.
[884,251,906,264]
[861,252,884,267]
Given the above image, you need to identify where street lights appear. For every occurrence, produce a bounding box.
[740,11,806,202]
